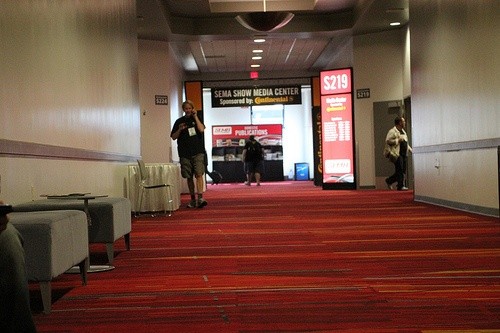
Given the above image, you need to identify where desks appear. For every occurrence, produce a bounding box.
[128,163,181,212]
[172,161,206,194]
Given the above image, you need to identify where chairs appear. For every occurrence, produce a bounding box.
[135,159,175,217]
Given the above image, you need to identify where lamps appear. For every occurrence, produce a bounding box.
[235,11,295,32]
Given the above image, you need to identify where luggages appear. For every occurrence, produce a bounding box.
[206,171,222,184]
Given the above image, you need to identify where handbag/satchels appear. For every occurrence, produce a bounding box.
[384,144,400,162]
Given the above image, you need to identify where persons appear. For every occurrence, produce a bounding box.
[384,117,413,190]
[170,101,208,209]
[242,135,262,185]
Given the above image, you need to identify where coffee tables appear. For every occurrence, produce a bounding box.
[46,194,115,274]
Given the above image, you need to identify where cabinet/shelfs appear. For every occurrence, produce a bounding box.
[211,145,284,183]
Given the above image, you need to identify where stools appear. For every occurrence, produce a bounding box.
[13,197,131,263]
[6,209,89,315]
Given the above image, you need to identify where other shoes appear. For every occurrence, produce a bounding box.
[197,200,208,208]
[385,180,392,190]
[186,200,196,208]
[256,181,260,186]
[401,186,409,190]
[245,179,251,186]
